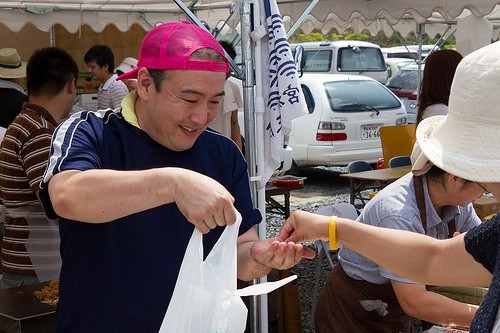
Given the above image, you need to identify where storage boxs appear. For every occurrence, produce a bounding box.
[473,198,498,216]
[77,94,98,111]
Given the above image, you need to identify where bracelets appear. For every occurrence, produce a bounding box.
[329,215,338,250]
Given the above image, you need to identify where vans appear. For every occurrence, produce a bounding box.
[289,40,389,85]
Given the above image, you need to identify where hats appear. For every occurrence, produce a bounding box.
[0,48,29,79]
[415,40,500,182]
[116,20,230,82]
[113,56,138,74]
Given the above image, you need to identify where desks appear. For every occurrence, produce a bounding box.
[0,279,55,333]
[265,184,305,220]
[339,165,412,216]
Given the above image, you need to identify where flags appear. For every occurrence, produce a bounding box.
[256,0,309,190]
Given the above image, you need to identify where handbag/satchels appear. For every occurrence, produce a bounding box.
[159,210,297,333]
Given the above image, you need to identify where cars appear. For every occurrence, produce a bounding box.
[379,46,423,83]
[230,71,408,176]
[383,64,425,124]
[394,44,440,62]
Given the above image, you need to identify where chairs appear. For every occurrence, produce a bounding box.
[348,160,376,207]
[388,156,411,168]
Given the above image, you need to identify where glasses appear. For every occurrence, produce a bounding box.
[476,181,491,195]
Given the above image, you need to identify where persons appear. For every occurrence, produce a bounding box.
[414,49,463,130]
[0,47,79,290]
[278,39,500,333]
[84,45,130,111]
[314,152,491,333]
[0,47,28,145]
[116,57,138,93]
[38,20,315,333]
[206,40,244,152]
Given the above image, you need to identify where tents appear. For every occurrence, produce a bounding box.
[0,0,268,333]
[284,6,500,110]
[274,0,500,28]
